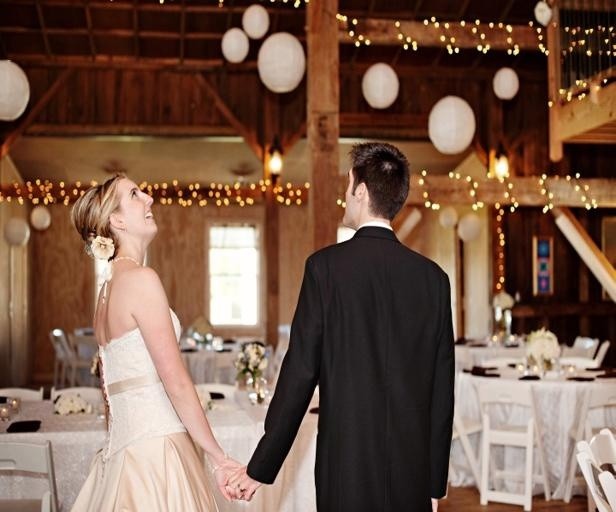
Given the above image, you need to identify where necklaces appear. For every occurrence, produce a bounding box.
[101,256,141,304]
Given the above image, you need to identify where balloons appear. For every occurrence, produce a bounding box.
[31,206,50,232]
[439,206,462,231]
[457,213,480,243]
[536,0,553,27]
[243,5,270,38]
[257,32,305,92]
[5,217,29,246]
[427,95,478,152]
[222,28,249,65]
[0,56,31,120]
[362,62,401,110]
[494,65,522,102]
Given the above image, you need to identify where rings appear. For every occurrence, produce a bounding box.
[238,484,245,492]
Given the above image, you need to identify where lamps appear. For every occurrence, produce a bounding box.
[268,133,286,183]
[494,139,511,181]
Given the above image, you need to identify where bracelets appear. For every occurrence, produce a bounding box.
[211,455,229,474]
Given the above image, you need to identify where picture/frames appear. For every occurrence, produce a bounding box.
[532,234,554,297]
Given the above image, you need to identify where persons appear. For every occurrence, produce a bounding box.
[69,174,245,512]
[226,143,455,512]
